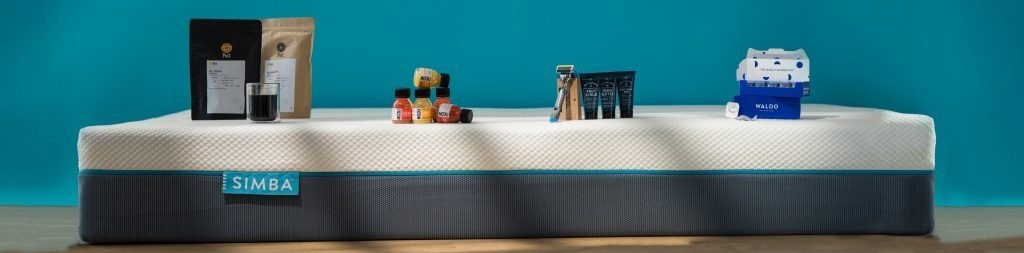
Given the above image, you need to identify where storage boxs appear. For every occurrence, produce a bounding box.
[726,49,810,119]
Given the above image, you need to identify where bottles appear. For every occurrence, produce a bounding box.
[434,103,473,123]
[412,89,433,124]
[433,87,451,123]
[391,88,412,124]
[413,68,450,87]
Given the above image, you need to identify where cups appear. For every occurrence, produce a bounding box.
[246,82,280,122]
